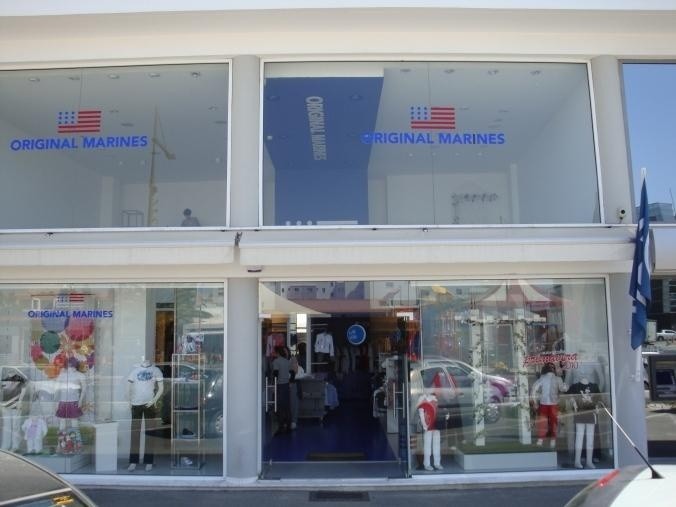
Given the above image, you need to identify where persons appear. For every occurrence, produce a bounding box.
[21,415,49,454]
[281,344,299,431]
[430,371,466,451]
[0,352,30,451]
[270,345,298,429]
[414,392,444,471]
[531,362,569,449]
[53,358,86,449]
[568,378,602,469]
[124,360,164,471]
[566,349,604,463]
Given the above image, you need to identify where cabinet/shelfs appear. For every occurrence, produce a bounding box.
[170,353,205,470]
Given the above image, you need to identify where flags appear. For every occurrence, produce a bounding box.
[628,178,654,350]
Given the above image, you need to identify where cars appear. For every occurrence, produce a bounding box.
[2,366,82,419]
[411,359,511,435]
[1,450,97,507]
[656,330,676,342]
[144,363,223,436]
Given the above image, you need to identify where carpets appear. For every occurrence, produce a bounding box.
[308,489,371,503]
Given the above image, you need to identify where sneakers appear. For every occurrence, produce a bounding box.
[181,456,192,465]
[182,428,194,435]
[434,464,444,470]
[291,423,296,428]
[425,465,433,471]
